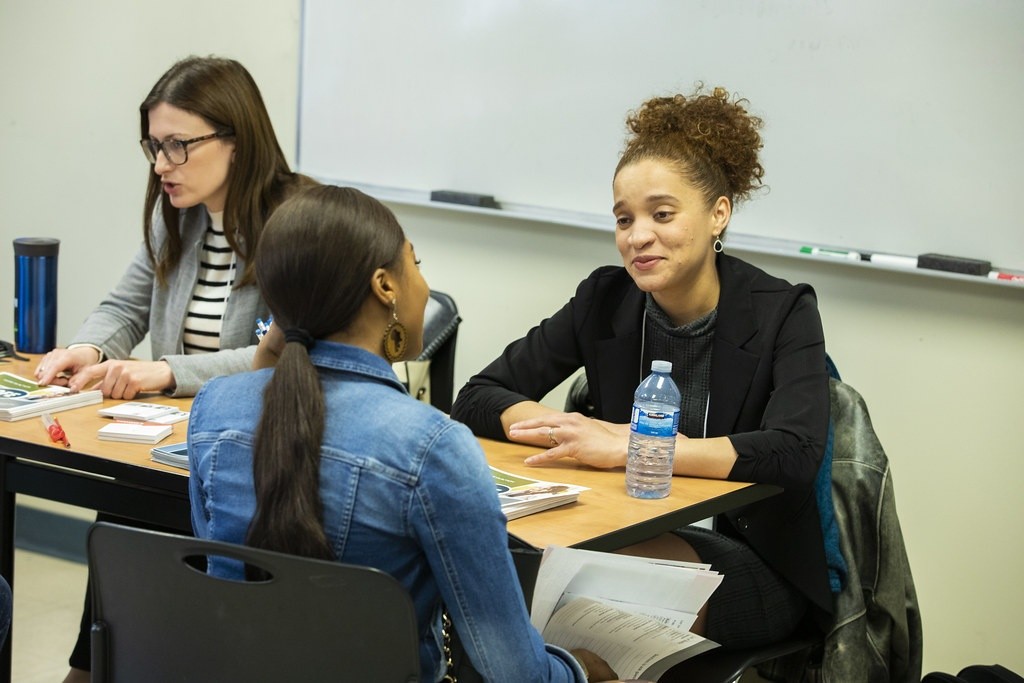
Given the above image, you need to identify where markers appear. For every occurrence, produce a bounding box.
[861,255,917,272]
[41,413,64,443]
[798,247,859,262]
[988,272,1023,284]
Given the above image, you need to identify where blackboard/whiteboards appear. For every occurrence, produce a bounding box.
[295,1,1023,289]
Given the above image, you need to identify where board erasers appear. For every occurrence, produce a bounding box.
[916,253,991,276]
[431,191,499,207]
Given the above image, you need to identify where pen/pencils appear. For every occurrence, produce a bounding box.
[54,416,71,448]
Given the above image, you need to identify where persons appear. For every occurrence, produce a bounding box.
[450,83,836,646]
[187,185,619,683]
[32,57,320,683]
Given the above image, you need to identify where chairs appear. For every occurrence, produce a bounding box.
[86,519,420,682]
[563,352,923,682]
[415,291,463,414]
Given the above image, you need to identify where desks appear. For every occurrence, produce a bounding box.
[0,355,785,682]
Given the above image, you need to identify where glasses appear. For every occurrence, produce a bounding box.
[140,129,228,166]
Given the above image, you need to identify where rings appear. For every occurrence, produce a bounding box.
[547,427,560,446]
[38,366,45,371]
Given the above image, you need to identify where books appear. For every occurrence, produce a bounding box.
[526,545,725,683]
[96,401,190,445]
[490,467,591,521]
[0,371,103,422]
[151,443,190,471]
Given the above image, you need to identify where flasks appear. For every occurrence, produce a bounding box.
[13,237,61,354]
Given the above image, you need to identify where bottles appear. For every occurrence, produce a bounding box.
[625,361,681,500]
